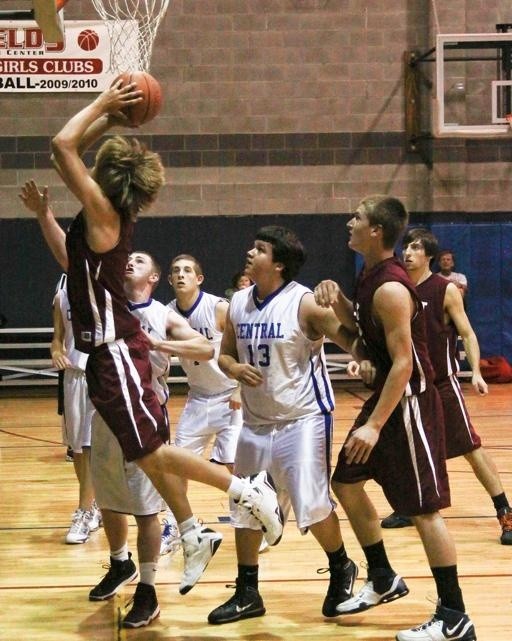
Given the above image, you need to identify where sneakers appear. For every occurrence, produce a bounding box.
[121,582,160,627]
[179,518,223,595]
[88,553,138,601]
[379,511,415,528]
[207,578,266,624]
[316,557,359,617]
[158,518,182,556]
[66,500,105,543]
[336,560,409,613]
[496,507,512,545]
[395,597,476,641]
[233,471,284,546]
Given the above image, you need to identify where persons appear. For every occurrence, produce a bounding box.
[18,177,512,641]
[48,73,285,595]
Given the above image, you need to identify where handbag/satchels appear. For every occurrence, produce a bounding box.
[479,356,512,383]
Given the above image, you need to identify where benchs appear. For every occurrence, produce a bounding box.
[0,329,368,385]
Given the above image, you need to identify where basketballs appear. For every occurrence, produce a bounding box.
[77,29,99,50]
[110,71,163,127]
